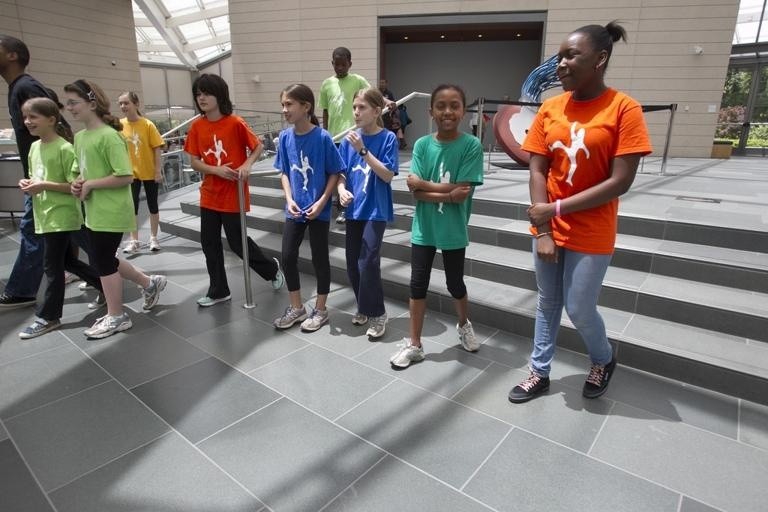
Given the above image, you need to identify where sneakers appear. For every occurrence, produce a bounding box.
[582,349,617,399]
[389,344,426,367]
[141,274,169,311]
[273,304,308,331]
[196,291,233,307]
[123,237,162,254]
[456,318,481,353]
[508,372,550,404]
[1,291,64,339]
[351,309,368,326]
[64,269,135,339]
[300,306,329,332]
[270,257,285,290]
[331,194,347,223]
[366,312,388,339]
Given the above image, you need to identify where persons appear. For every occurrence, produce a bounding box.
[391,100,408,138]
[388,82,486,370]
[332,89,400,338]
[317,45,370,225]
[0,32,106,311]
[377,79,406,150]
[58,78,168,338]
[504,21,653,405]
[18,93,108,341]
[113,90,166,255]
[181,73,284,310]
[271,82,347,333]
[471,95,486,149]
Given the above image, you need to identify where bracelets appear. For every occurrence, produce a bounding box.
[359,146,368,156]
[535,232,553,238]
[555,199,561,217]
[447,191,453,204]
